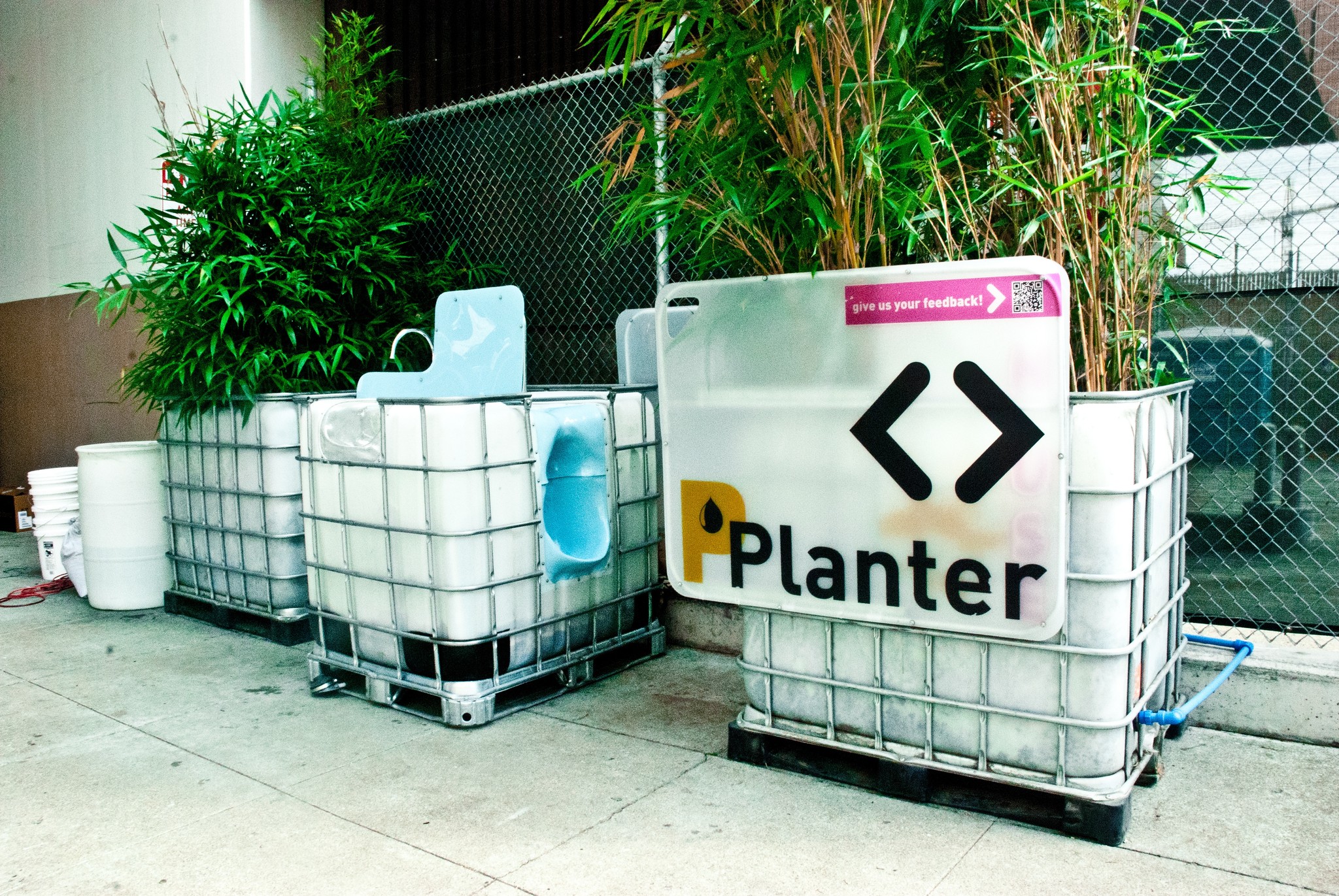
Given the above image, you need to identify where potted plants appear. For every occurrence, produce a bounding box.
[557,0,1281,846]
[55,7,509,649]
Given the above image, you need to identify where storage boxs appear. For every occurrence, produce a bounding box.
[0,488,35,533]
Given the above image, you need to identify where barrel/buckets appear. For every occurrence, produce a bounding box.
[27,466,81,580]
[75,441,175,611]
